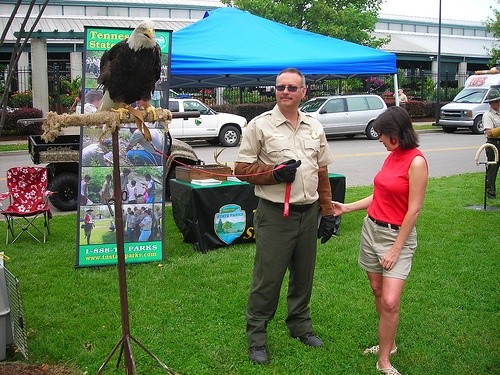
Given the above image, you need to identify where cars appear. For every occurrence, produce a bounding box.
[297,93,389,142]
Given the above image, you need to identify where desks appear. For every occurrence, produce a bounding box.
[171,172,347,253]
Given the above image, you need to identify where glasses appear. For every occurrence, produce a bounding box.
[377,132,386,138]
[275,86,303,92]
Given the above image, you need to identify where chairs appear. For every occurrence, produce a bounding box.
[0,166,54,245]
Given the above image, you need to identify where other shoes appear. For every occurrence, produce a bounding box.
[487,190,496,199]
[250,345,271,365]
[375,361,402,375]
[300,331,324,347]
[363,345,398,356]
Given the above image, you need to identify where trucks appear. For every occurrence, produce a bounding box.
[23,132,203,210]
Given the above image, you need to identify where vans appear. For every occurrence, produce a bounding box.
[439,65,500,135]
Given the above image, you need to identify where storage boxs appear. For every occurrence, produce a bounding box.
[176,165,233,182]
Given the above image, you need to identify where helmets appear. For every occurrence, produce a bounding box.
[483,88,500,102]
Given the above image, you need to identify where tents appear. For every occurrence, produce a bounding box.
[171,7,396,109]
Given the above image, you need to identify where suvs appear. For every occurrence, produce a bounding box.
[167,94,248,147]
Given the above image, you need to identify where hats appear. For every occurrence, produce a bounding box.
[122,168,131,173]
[86,208,94,212]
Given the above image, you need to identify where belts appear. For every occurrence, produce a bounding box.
[263,199,315,212]
[141,229,150,231]
[368,216,399,231]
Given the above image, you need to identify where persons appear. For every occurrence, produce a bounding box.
[234,68,334,365]
[482,89,500,199]
[80,55,167,245]
[393,89,407,110]
[73,91,81,112]
[331,106,428,375]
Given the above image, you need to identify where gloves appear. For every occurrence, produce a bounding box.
[318,215,337,245]
[274,159,301,184]
